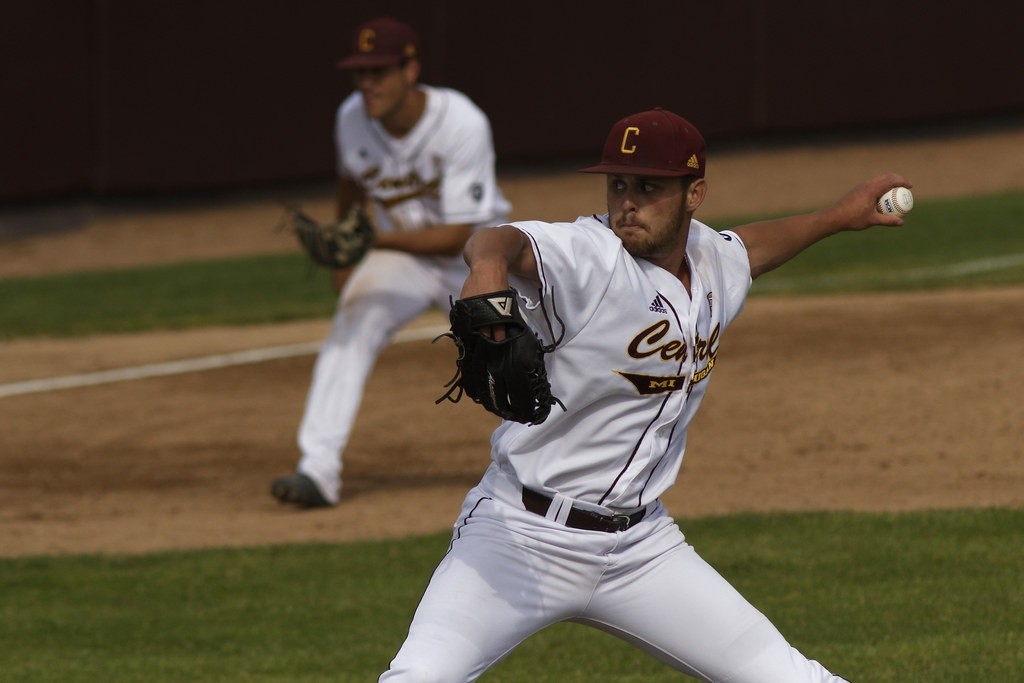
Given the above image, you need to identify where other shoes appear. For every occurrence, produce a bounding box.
[272,474,329,507]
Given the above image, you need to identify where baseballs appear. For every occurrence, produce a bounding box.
[875,187,914,217]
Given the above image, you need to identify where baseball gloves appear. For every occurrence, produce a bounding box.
[276,188,376,273]
[447,289,557,427]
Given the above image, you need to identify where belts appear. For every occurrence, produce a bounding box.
[521,486,647,533]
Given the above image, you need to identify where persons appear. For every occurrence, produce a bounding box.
[269,19,552,510]
[376,106,917,683]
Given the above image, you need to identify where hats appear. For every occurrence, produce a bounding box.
[338,16,417,71]
[576,107,706,178]
